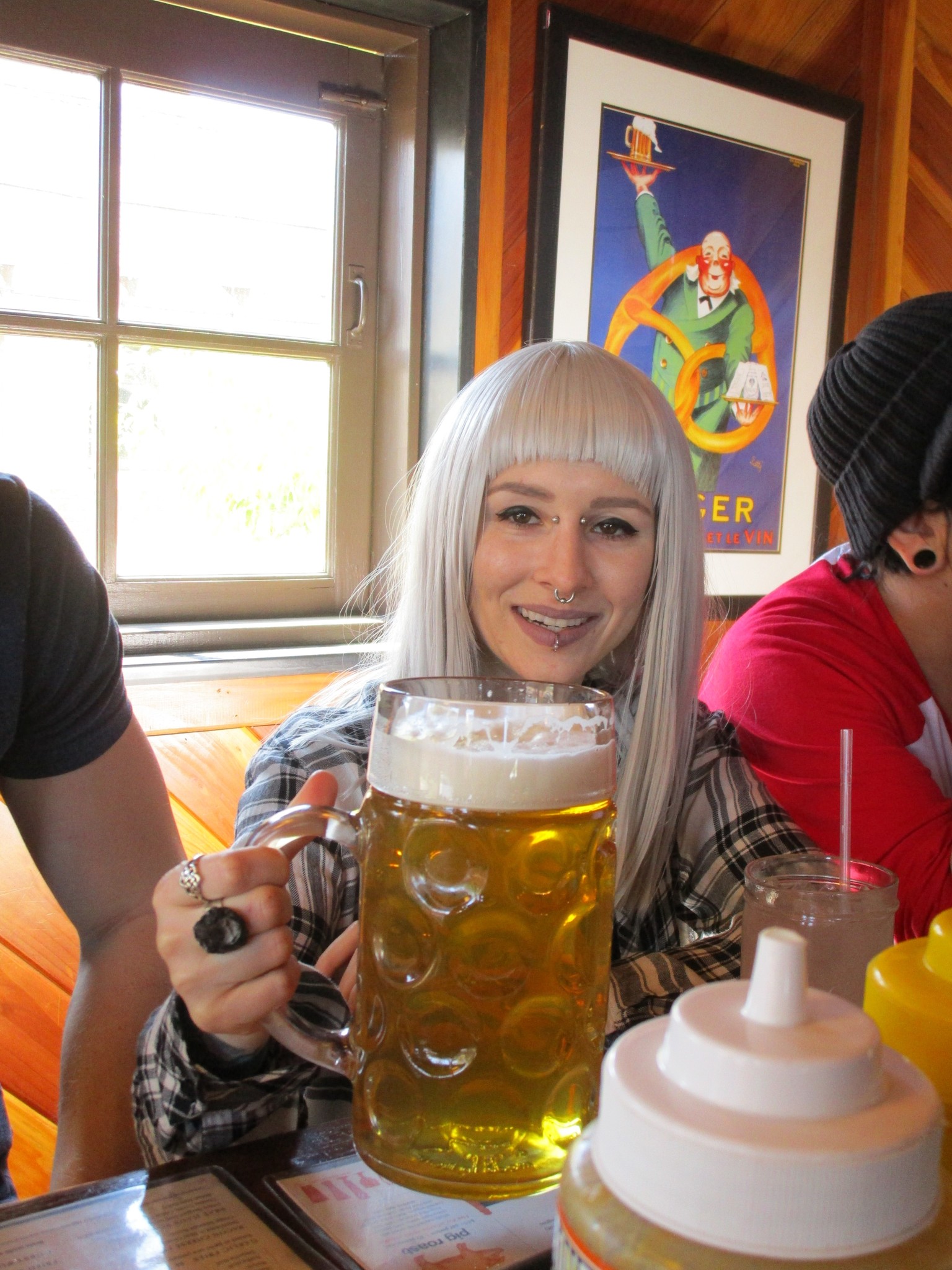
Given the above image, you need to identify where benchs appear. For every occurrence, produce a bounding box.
[0,672,358,1204]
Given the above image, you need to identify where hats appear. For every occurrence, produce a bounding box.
[807,293,952,561]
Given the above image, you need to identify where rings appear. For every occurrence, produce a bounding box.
[193,906,248,953]
[180,853,212,904]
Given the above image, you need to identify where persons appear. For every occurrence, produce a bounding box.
[0,476,189,1211]
[131,342,830,1168]
[694,289,951,946]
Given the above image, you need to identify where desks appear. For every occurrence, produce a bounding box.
[0,1116,561,1270]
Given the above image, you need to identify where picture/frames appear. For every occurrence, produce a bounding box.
[518,0,866,618]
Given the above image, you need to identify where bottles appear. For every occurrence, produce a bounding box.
[549,906,952,1270]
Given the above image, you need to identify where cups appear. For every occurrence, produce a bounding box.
[242,676,619,1202]
[742,852,901,1008]
